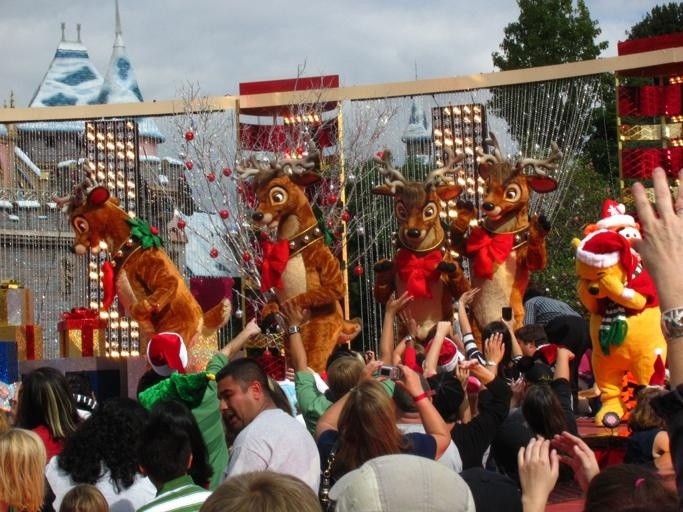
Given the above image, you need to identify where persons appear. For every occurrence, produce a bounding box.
[629,167,681,512]
[0,286,682,510]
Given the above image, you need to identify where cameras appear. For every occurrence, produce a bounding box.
[380,366,401,379]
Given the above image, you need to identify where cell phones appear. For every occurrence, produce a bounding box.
[502,306,512,321]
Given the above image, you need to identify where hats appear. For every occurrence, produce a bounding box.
[459,468,524,512]
[147,332,188,376]
[426,337,458,373]
[428,374,464,419]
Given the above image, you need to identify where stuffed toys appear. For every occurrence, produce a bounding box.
[584,200,641,251]
[50,180,231,355]
[570,229,667,426]
[452,130,552,357]
[370,154,472,338]
[237,155,360,376]
